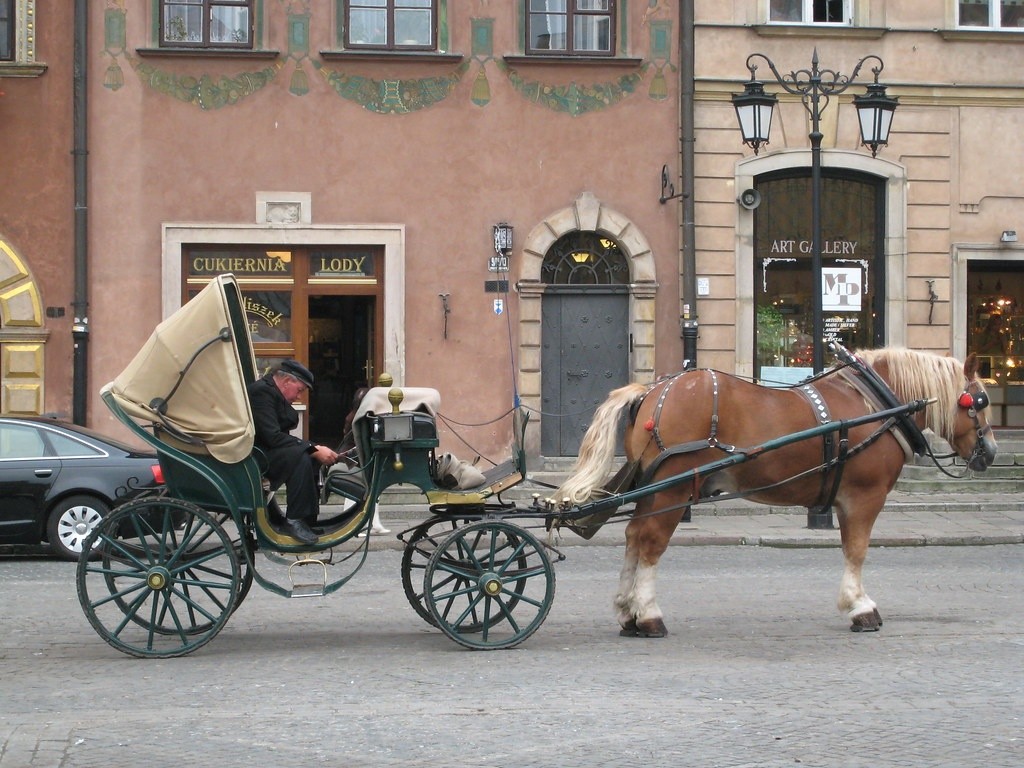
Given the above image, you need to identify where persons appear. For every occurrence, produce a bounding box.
[344,389,392,538]
[976,314,1010,379]
[246,359,337,543]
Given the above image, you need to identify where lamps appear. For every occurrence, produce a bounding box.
[1000,230,1018,242]
[493,222,514,257]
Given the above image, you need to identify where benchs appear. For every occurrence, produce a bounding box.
[367,386,439,448]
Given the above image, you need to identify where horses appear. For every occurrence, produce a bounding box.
[548,345,999,637]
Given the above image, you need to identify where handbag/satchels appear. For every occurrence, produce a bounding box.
[433,453,486,491]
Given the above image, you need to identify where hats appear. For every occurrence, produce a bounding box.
[280,360,314,389]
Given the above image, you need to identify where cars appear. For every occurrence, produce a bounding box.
[0,415,192,561]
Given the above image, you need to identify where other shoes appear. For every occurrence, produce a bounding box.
[369,526,391,534]
[357,532,367,538]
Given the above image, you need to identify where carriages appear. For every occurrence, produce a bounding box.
[74,269,997,661]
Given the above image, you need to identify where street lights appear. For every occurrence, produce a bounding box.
[730,51,900,375]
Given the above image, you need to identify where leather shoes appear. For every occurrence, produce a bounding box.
[278,517,323,544]
